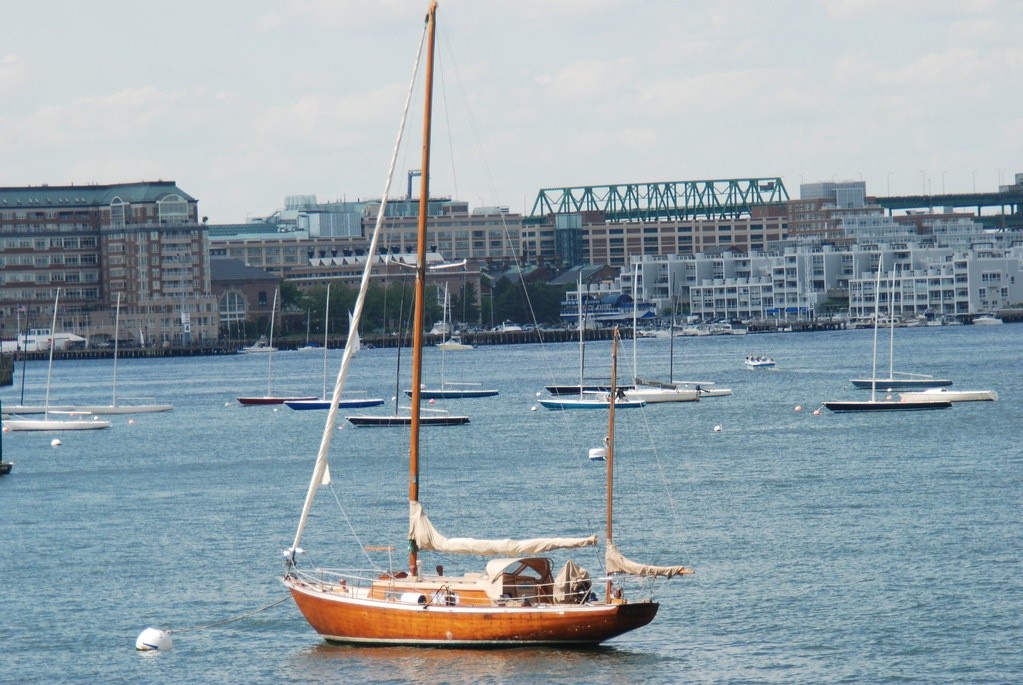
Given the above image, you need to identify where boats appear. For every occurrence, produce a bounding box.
[744,350,776,370]
[971,314,1009,327]
[821,254,952,415]
[899,387,999,404]
[838,257,966,331]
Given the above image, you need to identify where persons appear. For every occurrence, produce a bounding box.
[615,388,628,402]
[751,354,754,361]
[755,356,760,362]
[572,568,591,602]
[761,354,767,362]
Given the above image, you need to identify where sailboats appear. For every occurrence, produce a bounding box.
[342,275,471,429]
[0,297,75,416]
[69,292,176,417]
[237,290,319,407]
[274,0,701,651]
[434,280,475,352]
[404,279,500,401]
[235,288,280,354]
[534,263,733,411]
[0,285,114,433]
[849,262,954,391]
[294,306,327,352]
[282,283,385,411]
